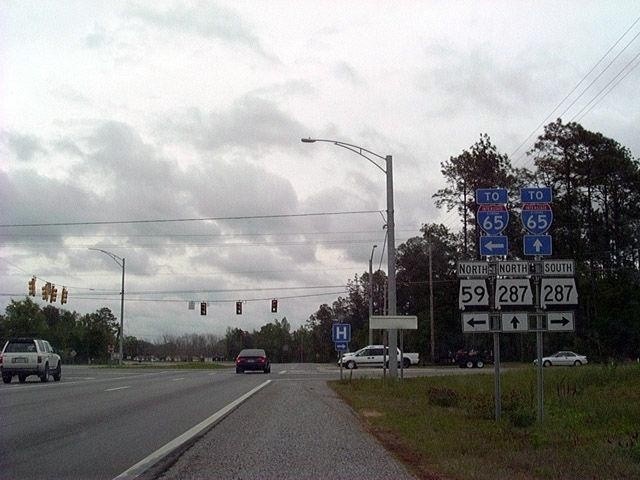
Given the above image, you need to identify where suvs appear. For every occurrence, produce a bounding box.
[454,350,484,368]
[0,338,63,386]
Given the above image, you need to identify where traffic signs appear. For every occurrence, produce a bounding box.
[520,200,553,235]
[477,203,511,236]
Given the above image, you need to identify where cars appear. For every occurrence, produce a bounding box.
[234,348,274,374]
[534,348,587,368]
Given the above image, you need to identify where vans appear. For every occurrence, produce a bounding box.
[338,346,404,369]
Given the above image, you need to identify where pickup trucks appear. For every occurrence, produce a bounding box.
[337,345,421,369]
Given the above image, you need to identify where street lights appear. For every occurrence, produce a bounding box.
[86,247,126,365]
[367,237,379,347]
[296,133,403,376]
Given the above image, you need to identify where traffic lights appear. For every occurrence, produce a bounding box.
[271,300,278,312]
[25,278,69,306]
[200,303,207,316]
[235,301,243,315]
